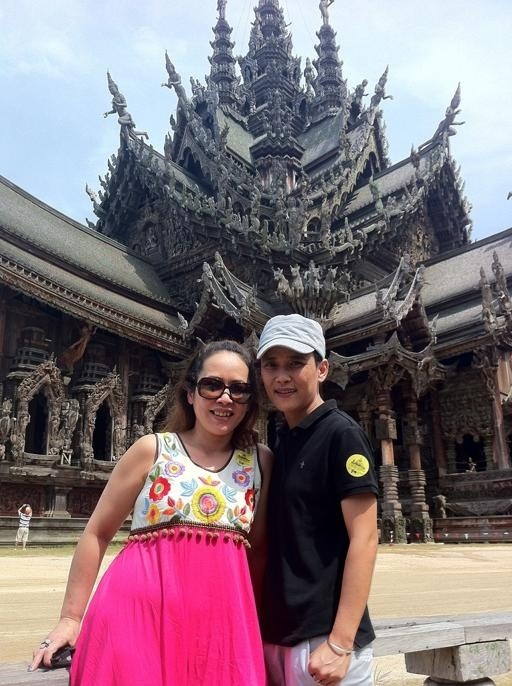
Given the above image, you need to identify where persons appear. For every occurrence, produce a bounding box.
[15,503,33,551]
[256,315,379,686]
[28,340,273,686]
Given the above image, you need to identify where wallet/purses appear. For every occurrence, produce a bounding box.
[36,643,76,671]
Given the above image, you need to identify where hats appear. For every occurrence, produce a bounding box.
[255,313,326,362]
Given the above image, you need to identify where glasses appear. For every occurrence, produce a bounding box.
[195,377,256,404]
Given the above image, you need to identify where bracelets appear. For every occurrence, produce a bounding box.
[327,636,352,654]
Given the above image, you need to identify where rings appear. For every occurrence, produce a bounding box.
[314,675,320,682]
[39,639,51,649]
